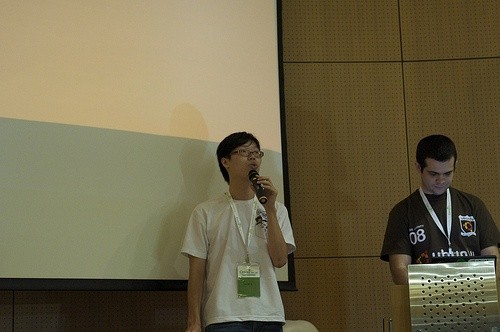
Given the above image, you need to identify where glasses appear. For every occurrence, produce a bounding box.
[231,148,264,158]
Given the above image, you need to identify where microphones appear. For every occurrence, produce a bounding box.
[249,169,267,204]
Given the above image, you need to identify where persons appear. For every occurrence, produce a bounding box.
[180,132,296,332]
[381,134,500,285]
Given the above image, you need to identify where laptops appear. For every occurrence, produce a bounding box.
[432,256,497,271]
